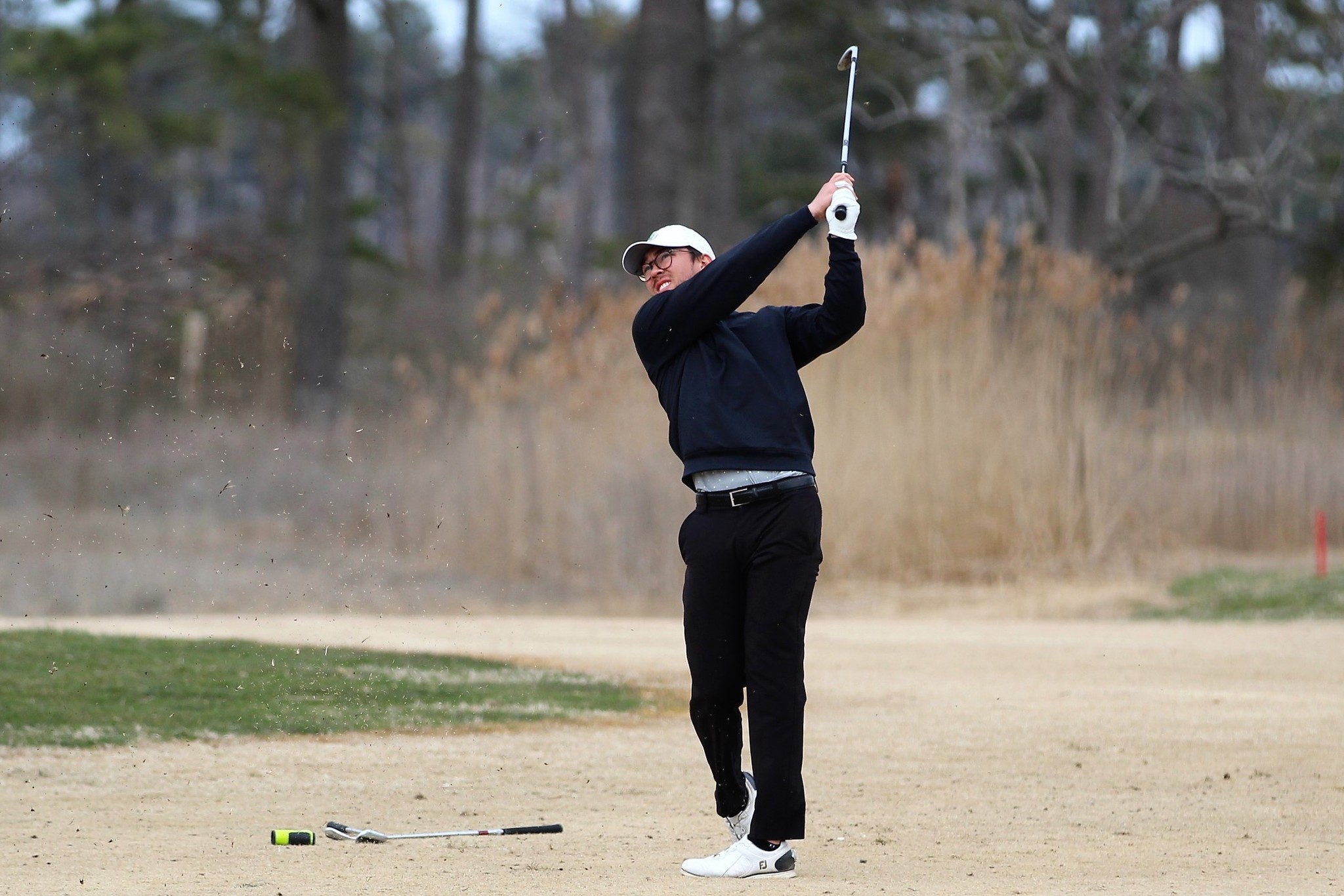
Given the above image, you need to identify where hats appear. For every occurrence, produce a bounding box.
[622,224,716,277]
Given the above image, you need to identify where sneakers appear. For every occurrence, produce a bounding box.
[680,833,798,880]
[725,771,757,844]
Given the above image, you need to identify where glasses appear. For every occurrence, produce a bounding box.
[636,249,699,282]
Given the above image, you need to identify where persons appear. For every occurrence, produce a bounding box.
[622,171,866,881]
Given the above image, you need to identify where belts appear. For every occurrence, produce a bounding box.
[696,475,815,508]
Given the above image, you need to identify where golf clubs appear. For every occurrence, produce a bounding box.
[324,823,564,842]
[835,44,859,221]
[321,818,388,845]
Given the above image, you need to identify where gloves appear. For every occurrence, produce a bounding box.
[825,181,861,240]
